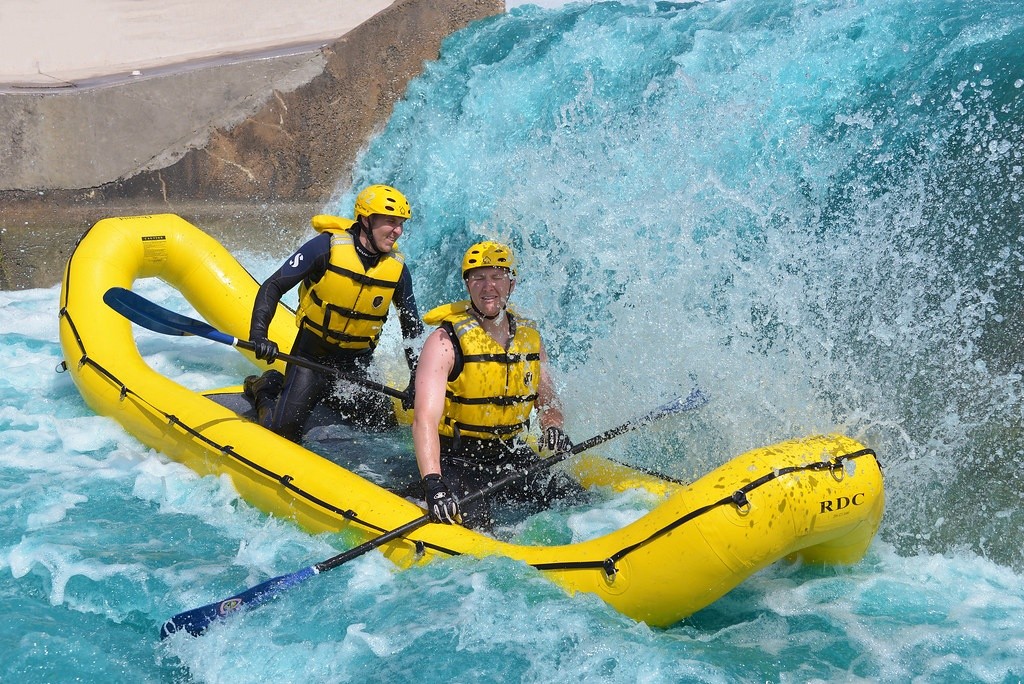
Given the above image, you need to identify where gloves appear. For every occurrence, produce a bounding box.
[539,427,573,453]
[250,337,279,365]
[401,386,416,411]
[422,473,462,523]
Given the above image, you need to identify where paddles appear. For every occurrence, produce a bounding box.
[99,284,411,400]
[158,343,796,650]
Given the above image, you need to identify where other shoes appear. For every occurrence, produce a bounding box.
[244,369,284,398]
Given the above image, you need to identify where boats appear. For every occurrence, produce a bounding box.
[57,211,886,632]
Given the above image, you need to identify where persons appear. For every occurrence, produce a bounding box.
[243,183,424,439]
[411,240,593,526]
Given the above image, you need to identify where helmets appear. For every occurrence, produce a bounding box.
[355,185,411,221]
[462,241,518,279]
